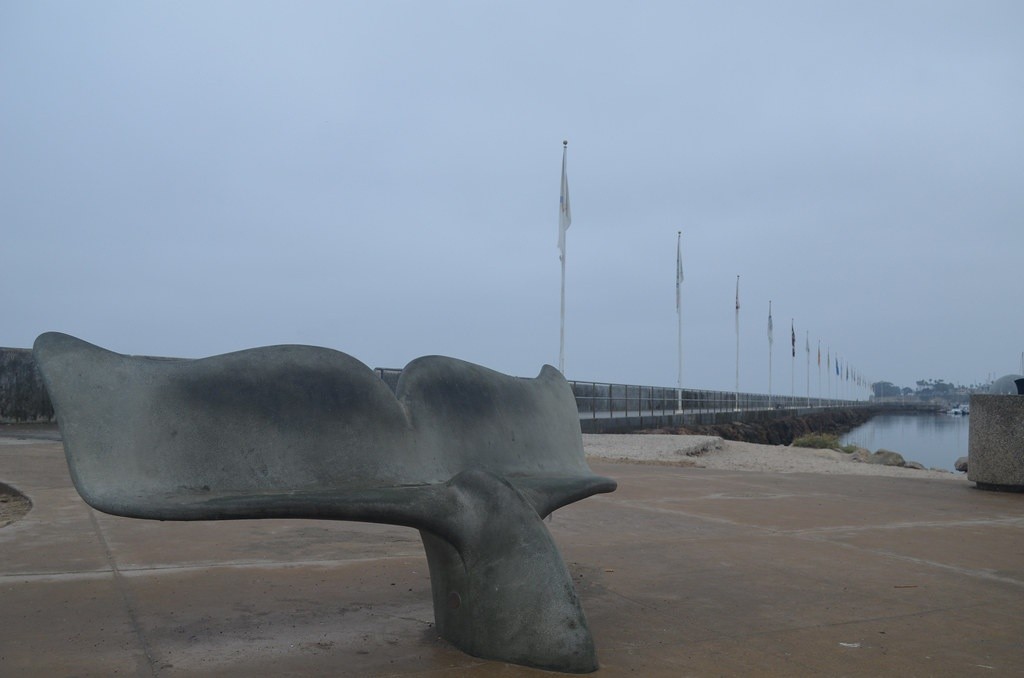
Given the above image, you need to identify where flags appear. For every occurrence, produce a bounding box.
[734,279,741,333]
[555,147,572,262]
[826,350,832,375]
[835,355,874,392]
[805,333,811,361]
[817,344,821,369]
[790,322,796,359]
[767,303,774,344]
[675,238,684,312]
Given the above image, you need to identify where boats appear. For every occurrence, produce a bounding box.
[950,405,969,416]
